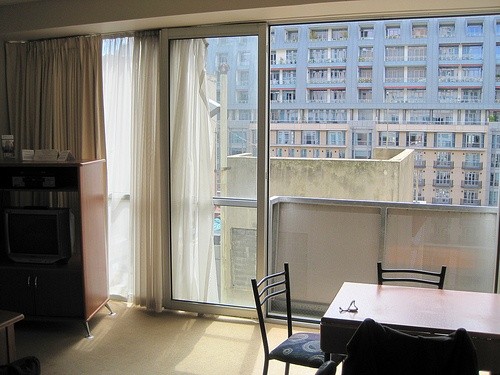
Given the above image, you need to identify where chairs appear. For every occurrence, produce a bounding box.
[376,260,448,289]
[343,317,478,375]
[252,262,326,375]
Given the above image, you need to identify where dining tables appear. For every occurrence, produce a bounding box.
[320,282,499,375]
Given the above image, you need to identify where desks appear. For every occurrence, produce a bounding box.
[0,309,26,366]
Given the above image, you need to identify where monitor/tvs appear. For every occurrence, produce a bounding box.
[4,209,71,263]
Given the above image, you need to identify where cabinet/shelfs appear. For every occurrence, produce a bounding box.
[0,154,117,344]
